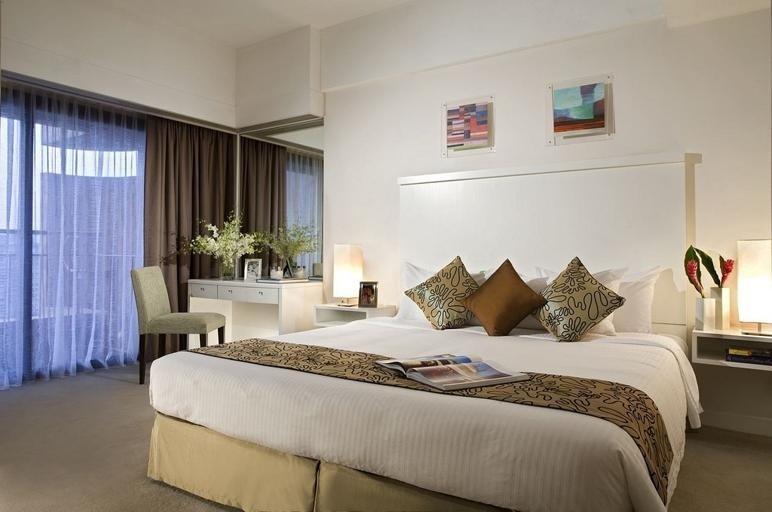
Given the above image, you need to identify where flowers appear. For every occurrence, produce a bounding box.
[251,213,321,280]
[158,210,256,267]
[694,245,736,287]
[681,242,712,298]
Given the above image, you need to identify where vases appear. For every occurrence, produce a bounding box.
[281,254,298,280]
[216,259,238,281]
[710,286,732,331]
[694,297,717,333]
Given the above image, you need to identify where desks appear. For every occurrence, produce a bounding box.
[185,274,323,350]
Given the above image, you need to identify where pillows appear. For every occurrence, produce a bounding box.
[379,253,671,344]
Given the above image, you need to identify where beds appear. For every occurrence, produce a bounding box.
[143,152,708,512]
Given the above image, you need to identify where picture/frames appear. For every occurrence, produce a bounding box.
[541,71,616,148]
[358,280,379,308]
[440,96,498,159]
[243,257,263,283]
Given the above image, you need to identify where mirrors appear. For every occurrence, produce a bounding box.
[238,116,325,279]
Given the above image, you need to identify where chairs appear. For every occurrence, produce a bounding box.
[130,264,226,386]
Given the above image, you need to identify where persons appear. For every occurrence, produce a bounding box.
[246,262,259,280]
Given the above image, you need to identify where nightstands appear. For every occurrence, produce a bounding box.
[312,303,397,327]
[692,328,772,373]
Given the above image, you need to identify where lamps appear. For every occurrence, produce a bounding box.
[734,238,772,337]
[330,243,367,307]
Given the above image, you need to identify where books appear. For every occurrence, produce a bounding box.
[375,353,530,390]
[725,345,772,365]
